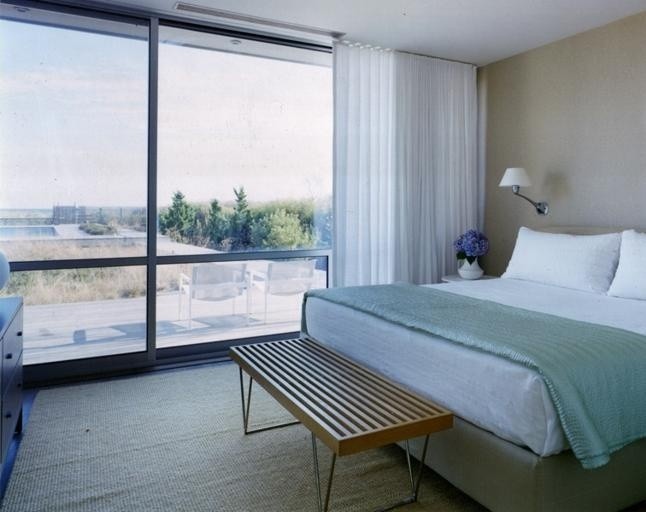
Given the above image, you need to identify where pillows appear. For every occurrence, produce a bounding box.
[605,227,646,303]
[498,224,623,294]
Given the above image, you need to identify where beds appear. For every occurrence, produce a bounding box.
[299,219,646,511]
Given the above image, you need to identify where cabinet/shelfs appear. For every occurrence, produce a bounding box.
[0,289,25,488]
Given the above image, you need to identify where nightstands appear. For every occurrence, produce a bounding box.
[440,271,499,283]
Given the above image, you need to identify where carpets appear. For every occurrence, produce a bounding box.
[2,355,485,511]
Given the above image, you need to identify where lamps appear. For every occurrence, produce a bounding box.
[497,164,550,219]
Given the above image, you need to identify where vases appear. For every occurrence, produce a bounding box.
[457,255,486,280]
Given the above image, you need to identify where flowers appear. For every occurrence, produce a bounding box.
[451,227,492,267]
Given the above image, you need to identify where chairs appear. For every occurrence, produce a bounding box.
[175,261,251,331]
[250,257,318,328]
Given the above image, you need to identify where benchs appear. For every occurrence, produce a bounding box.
[228,335,458,512]
